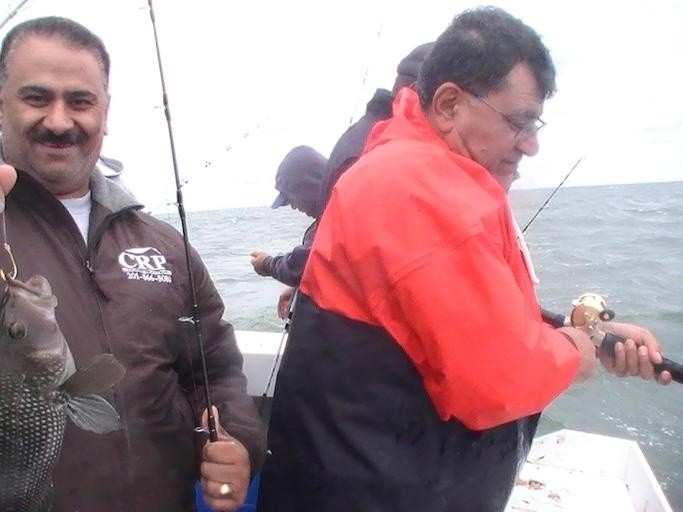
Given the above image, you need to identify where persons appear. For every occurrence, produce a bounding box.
[253,5,675,512]
[0,13,269,512]
[249,143,329,322]
[313,39,439,233]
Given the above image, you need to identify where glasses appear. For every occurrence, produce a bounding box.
[451,81,547,142]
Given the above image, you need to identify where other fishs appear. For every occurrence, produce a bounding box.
[0,271,128,512]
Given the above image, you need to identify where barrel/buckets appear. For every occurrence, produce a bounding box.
[193,473,263,512]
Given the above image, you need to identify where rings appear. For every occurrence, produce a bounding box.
[219,482,232,498]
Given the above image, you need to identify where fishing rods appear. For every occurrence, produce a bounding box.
[0,0,216,483]
[522,156,582,236]
[258,28,384,414]
[541,293,682,383]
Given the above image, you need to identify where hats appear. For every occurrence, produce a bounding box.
[271,145,328,210]
